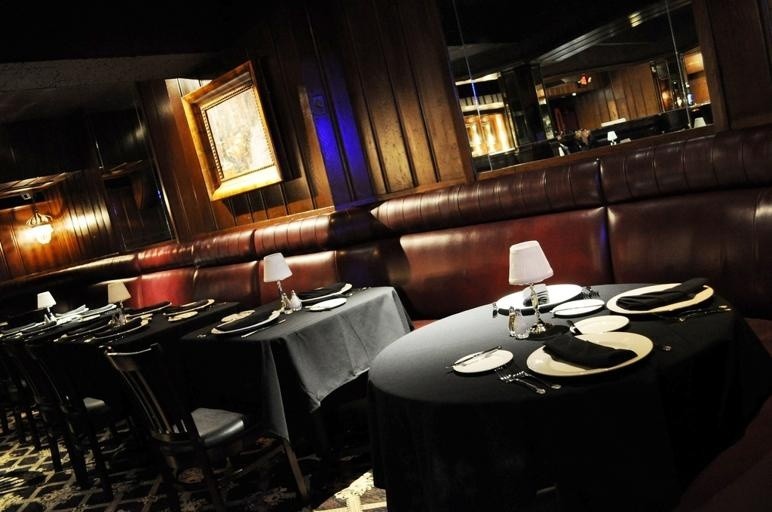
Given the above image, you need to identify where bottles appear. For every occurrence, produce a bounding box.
[280,293,293,314]
[508,306,516,336]
[290,290,302,311]
[514,309,530,339]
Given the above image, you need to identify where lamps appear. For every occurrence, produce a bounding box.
[26,201,52,244]
[509,240,554,339]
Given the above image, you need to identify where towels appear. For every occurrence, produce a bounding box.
[523,283,549,306]
[617,264,710,311]
[543,332,637,369]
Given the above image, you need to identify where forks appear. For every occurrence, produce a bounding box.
[587,286,600,298]
[507,359,562,390]
[495,365,547,396]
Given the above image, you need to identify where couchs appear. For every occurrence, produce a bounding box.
[600,122,771,511]
[253,156,613,329]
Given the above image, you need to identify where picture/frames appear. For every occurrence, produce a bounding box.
[180,59,293,203]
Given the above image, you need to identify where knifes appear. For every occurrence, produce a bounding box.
[241,319,287,338]
[445,345,502,369]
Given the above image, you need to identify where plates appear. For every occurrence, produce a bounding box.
[526,331,653,377]
[93,318,149,340]
[496,284,582,309]
[124,302,172,317]
[570,315,629,332]
[553,298,605,315]
[607,283,714,314]
[310,298,346,310]
[163,298,215,316]
[168,311,198,322]
[301,283,352,302]
[211,310,280,334]
[452,349,513,373]
